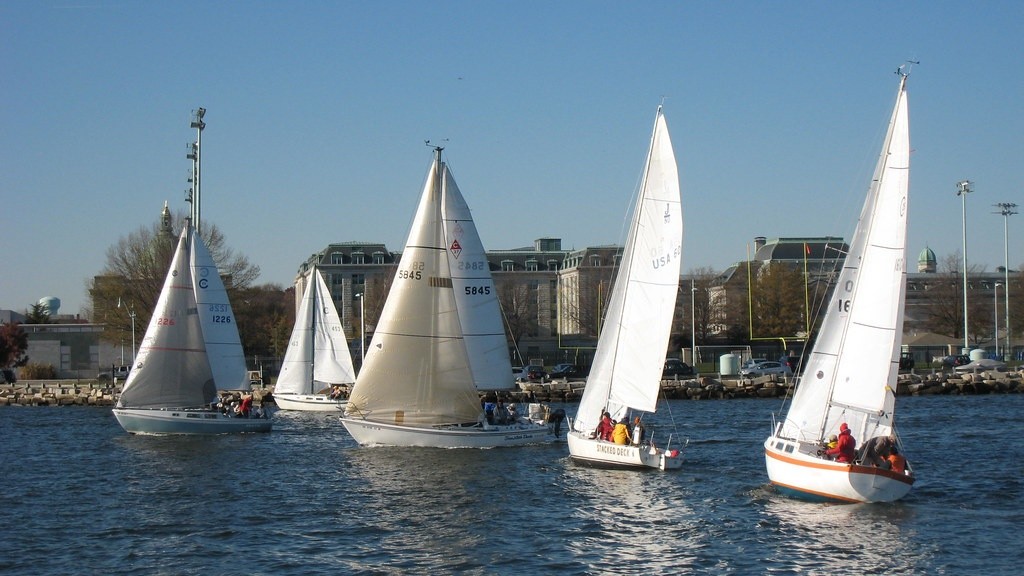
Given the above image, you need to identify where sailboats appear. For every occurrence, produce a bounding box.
[566,94,686,471]
[110,215,274,435]
[763,59,922,502]
[338,140,553,447]
[272,262,356,411]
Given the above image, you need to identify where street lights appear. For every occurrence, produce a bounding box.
[355,292,365,364]
[994,282,1002,360]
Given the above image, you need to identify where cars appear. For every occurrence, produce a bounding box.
[743,358,768,368]
[778,355,805,373]
[742,361,792,379]
[662,359,693,376]
[98,365,132,382]
[512,364,585,383]
[941,355,971,370]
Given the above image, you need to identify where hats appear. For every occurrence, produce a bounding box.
[885,446,898,454]
[624,414,629,419]
[890,434,897,444]
[828,435,837,441]
[603,412,610,418]
[840,423,849,431]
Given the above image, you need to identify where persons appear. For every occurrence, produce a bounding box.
[858,433,897,468]
[254,401,269,419]
[594,412,645,447]
[331,386,343,400]
[492,399,519,426]
[219,391,253,418]
[826,435,839,461]
[874,445,906,475]
[821,423,857,464]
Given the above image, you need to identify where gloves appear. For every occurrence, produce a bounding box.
[817,449,826,456]
